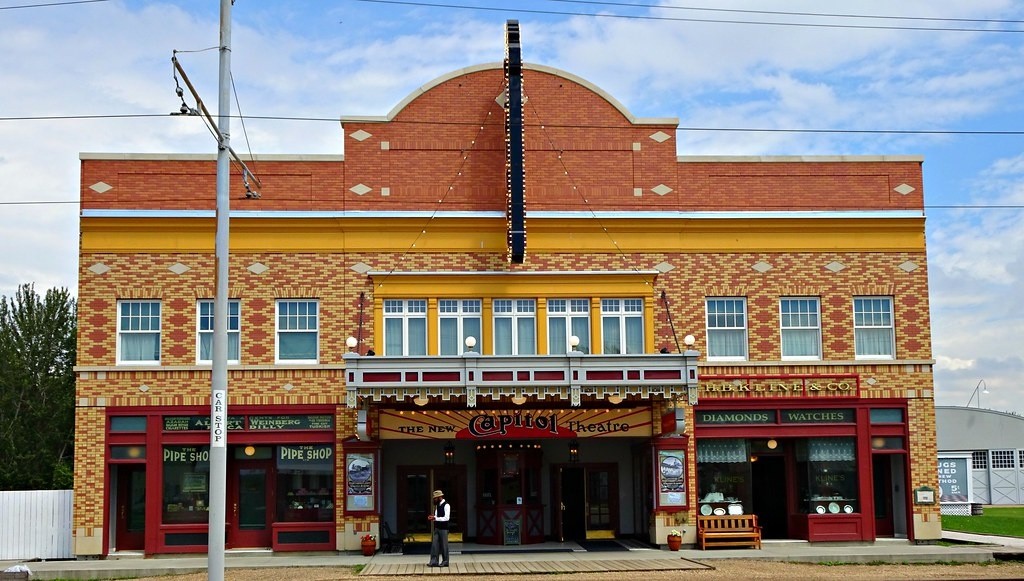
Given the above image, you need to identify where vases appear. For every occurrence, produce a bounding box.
[360,539,377,556]
[667,535,681,551]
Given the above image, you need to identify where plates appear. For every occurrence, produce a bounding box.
[701,505,712,515]
[728,504,743,515]
[828,502,840,513]
[844,504,853,513]
[714,508,727,516]
[816,506,826,514]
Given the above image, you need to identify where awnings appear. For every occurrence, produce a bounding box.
[809,438,856,461]
[698,438,751,463]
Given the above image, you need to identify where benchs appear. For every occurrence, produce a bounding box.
[697,514,761,551]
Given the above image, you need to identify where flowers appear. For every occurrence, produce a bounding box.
[360,534,376,541]
[670,529,687,537]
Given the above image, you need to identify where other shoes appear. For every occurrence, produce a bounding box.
[439,562,449,567]
[427,561,439,567]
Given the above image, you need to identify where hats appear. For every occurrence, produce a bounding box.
[433,490,444,499]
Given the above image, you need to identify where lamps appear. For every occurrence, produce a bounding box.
[684,335,695,350]
[767,437,777,449]
[570,336,580,351]
[464,336,476,352]
[245,445,255,455]
[346,337,358,352]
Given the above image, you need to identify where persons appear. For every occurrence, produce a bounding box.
[427,489,451,567]
